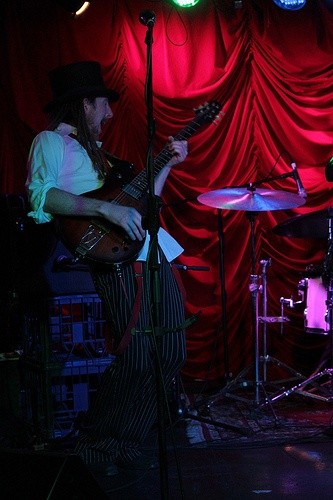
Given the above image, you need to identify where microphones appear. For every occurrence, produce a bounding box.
[139,10,155,28]
[291,159,307,198]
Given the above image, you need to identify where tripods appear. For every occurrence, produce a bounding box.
[199,183,333,425]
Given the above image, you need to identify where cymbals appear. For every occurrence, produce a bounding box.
[197,187,306,211]
[272,208,333,240]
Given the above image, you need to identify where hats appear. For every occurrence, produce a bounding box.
[41,61,120,113]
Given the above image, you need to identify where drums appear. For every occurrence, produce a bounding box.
[299,278,330,335]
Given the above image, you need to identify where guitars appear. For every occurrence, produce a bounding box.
[51,98,225,264]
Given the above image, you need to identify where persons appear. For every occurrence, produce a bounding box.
[25,61,188,476]
